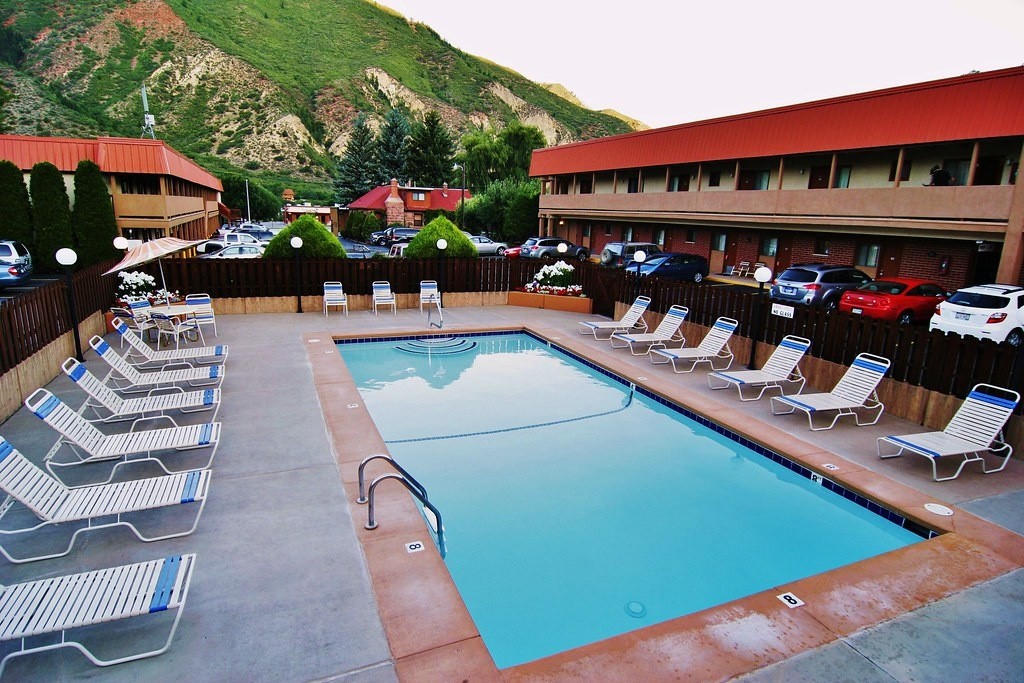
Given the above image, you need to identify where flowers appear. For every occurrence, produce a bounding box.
[515,261,586,296]
[115,271,185,310]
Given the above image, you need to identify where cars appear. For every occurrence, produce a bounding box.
[840,276,951,326]
[624,253,709,287]
[462,231,521,260]
[197,244,265,259]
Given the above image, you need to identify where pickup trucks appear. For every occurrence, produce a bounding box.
[207,233,270,251]
[0,260,32,295]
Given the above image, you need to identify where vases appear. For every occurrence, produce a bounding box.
[535,288,582,297]
[116,298,127,308]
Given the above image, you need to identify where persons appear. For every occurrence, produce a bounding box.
[929,165,955,186]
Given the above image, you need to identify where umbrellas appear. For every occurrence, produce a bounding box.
[102,236,210,309]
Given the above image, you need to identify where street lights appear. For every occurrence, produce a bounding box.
[113,236,128,297]
[557,242,567,260]
[453,162,465,230]
[746,267,772,370]
[437,239,448,307]
[55,248,87,362]
[632,250,646,329]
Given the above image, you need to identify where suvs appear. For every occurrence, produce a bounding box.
[769,262,873,316]
[0,240,32,267]
[219,224,274,241]
[929,284,1024,349]
[520,237,590,262]
[599,241,662,271]
[371,228,391,246]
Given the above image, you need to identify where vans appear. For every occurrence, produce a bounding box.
[385,227,420,244]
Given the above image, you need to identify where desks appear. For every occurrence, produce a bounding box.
[142,306,198,347]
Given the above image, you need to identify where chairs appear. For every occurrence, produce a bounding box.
[707,335,811,401]
[372,281,396,315]
[730,261,765,279]
[649,317,738,373]
[0,294,228,678]
[323,281,348,317]
[610,305,689,355]
[877,383,1020,481]
[420,280,441,313]
[770,353,891,430]
[578,295,651,341]
